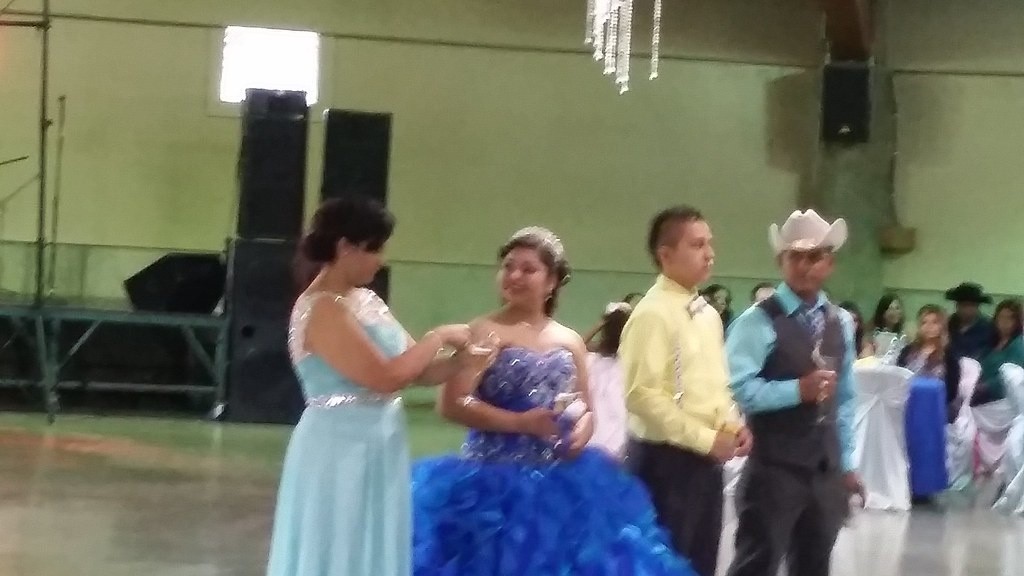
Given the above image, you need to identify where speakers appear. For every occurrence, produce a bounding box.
[819,61,871,143]
[223,87,393,424]
[122,252,226,316]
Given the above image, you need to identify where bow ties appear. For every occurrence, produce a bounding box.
[686,295,706,318]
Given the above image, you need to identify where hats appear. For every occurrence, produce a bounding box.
[944,283,992,305]
[769,208,848,258]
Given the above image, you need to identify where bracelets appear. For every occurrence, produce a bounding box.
[426,331,444,353]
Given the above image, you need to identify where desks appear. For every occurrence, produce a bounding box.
[910,376,948,505]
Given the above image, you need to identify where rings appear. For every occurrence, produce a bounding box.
[820,378,828,388]
[819,395,828,404]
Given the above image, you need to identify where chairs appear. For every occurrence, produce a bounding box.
[839,359,915,514]
[943,357,1024,519]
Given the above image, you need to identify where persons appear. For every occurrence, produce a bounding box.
[267,194,495,576]
[412,226,694,576]
[584,205,1024,576]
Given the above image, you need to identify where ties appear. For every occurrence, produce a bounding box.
[798,305,823,338]
